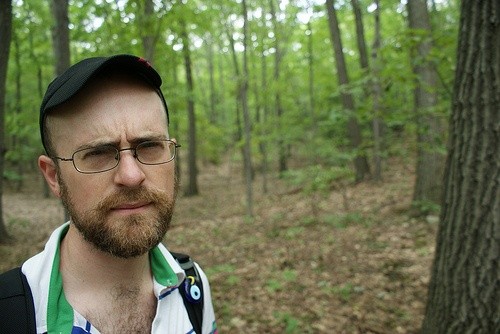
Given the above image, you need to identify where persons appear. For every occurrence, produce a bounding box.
[0,55,218,334]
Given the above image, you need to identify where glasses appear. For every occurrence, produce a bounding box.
[50,139,182,174]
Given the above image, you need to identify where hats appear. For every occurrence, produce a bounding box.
[40,54,162,114]
